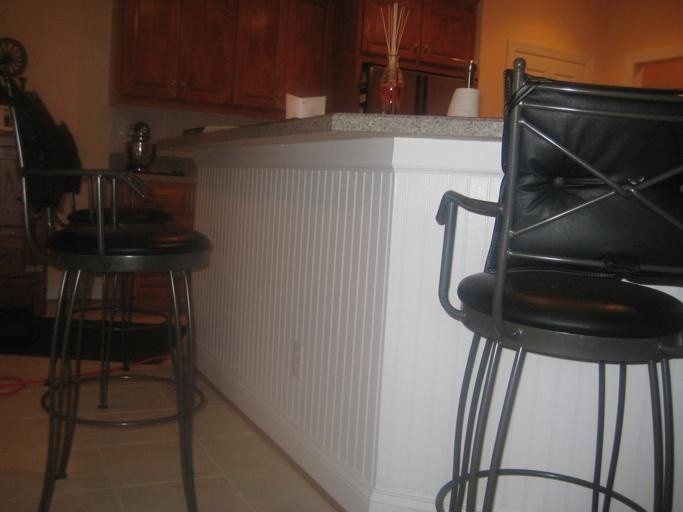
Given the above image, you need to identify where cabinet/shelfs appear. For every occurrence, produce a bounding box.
[331,2,480,116]
[105,173,194,313]
[109,0,231,111]
[233,0,338,114]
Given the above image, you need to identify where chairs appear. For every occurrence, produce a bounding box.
[433,54,683,512]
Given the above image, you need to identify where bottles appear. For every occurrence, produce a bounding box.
[379,55,404,113]
